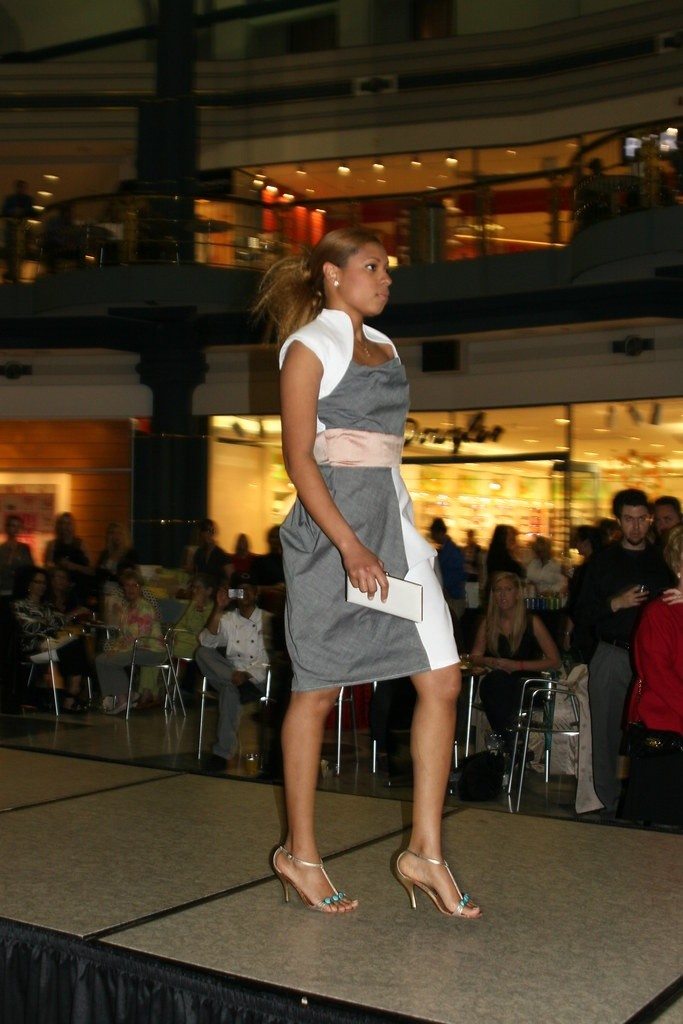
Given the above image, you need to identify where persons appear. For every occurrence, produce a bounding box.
[431,487,682,815]
[95,572,165,716]
[464,529,482,582]
[527,537,568,594]
[258,227,482,920]
[0,513,288,772]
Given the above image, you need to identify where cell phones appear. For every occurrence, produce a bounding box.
[227,589,244,599]
[640,585,644,594]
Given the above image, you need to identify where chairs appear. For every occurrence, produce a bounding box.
[260,665,361,777]
[125,626,187,720]
[197,664,274,769]
[507,665,588,813]
[21,622,95,717]
[464,668,555,783]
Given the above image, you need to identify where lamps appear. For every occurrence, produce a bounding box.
[649,403,662,426]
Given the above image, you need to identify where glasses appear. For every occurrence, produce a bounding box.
[124,583,139,588]
[31,579,47,586]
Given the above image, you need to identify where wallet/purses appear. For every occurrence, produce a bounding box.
[346,572,423,623]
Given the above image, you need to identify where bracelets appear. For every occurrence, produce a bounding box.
[520,661,525,669]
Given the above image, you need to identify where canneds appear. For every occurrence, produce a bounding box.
[525,597,560,610]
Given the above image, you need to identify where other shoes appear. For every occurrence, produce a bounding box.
[209,756,227,773]
[237,679,262,704]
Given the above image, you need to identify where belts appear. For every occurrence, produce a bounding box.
[601,635,633,652]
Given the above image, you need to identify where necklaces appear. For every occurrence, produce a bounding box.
[356,339,370,356]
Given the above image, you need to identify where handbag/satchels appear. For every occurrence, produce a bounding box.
[459,745,502,801]
[629,723,682,761]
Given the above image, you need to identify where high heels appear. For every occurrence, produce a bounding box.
[106,702,128,717]
[272,845,358,912]
[396,849,483,919]
[129,693,143,705]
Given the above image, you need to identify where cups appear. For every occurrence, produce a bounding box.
[636,585,648,595]
[460,653,472,668]
[246,752,259,773]
